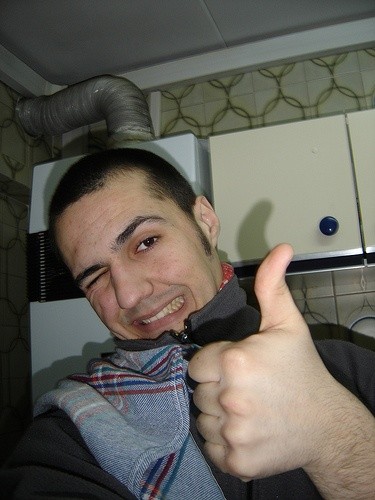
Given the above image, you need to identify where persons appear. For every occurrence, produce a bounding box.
[2,147,375,500]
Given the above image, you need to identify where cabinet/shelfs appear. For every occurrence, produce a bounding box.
[207,105,375,276]
